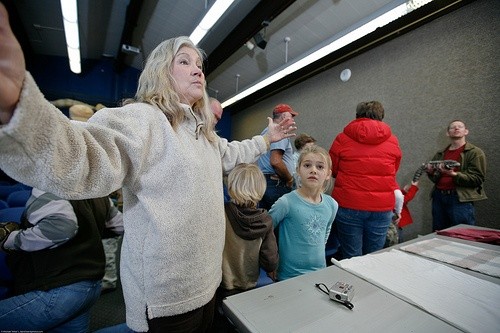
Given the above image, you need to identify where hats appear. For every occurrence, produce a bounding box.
[68,104,94,123]
[272,104,299,116]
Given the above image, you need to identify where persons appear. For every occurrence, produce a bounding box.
[0,98,140,329]
[386,163,425,236]
[0,1,296,333]
[204,96,226,128]
[294,131,318,154]
[265,143,339,280]
[330,96,400,258]
[254,92,297,202]
[219,161,283,299]
[427,118,491,231]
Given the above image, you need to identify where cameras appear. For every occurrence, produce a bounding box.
[328,281,355,303]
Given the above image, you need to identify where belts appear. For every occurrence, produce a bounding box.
[433,189,456,195]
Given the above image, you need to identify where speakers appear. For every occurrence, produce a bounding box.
[253,32,267,49]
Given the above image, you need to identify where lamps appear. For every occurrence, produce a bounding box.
[252,22,268,49]
[59,0,81,74]
[190,0,239,47]
[220,0,431,109]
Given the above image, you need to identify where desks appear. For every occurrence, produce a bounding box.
[221,224,500,333]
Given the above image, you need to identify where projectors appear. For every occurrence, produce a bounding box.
[121,44,140,55]
